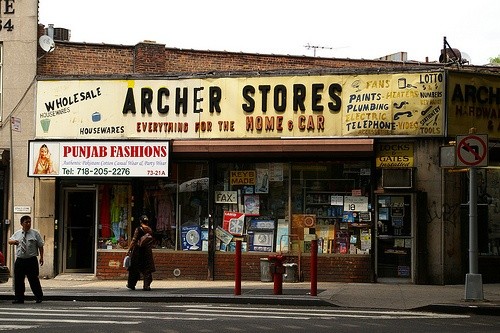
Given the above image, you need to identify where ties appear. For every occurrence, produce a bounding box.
[20,231,29,253]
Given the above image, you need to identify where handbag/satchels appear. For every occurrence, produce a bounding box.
[123,254,130,268]
[136,226,154,250]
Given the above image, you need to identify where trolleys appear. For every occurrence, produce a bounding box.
[279,233,304,282]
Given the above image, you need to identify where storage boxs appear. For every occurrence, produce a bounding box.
[291,214,371,254]
[180,193,289,254]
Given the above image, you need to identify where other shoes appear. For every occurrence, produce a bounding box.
[143,286,151,291]
[126,284,135,290]
[12,300,24,304]
[36,298,42,303]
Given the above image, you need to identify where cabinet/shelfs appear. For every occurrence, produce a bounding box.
[99,185,132,248]
[269,180,357,222]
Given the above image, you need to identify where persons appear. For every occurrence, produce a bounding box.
[125,215,155,290]
[34,144,57,175]
[8,215,45,305]
